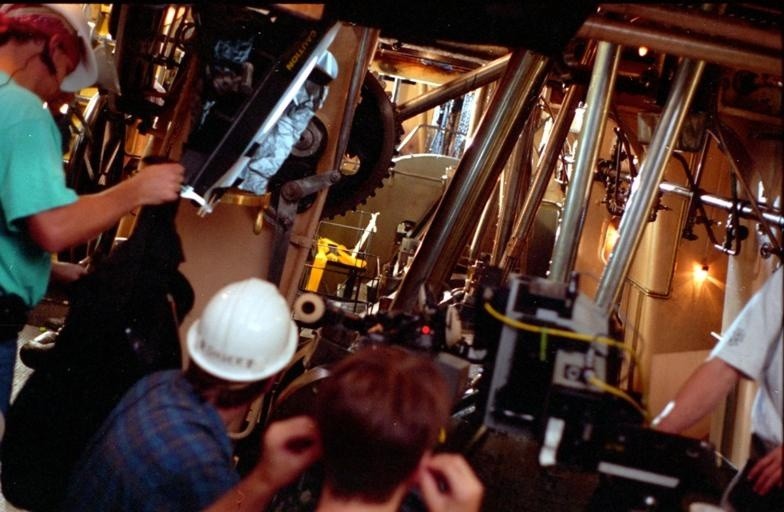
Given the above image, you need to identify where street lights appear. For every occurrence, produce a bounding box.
[0,197,195,512]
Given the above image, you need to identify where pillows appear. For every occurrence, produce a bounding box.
[0,0,99,93]
[186,278,299,384]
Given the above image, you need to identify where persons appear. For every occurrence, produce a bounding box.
[650,264,783,512]
[62,279,298,511]
[0,0,185,444]
[202,342,484,510]
